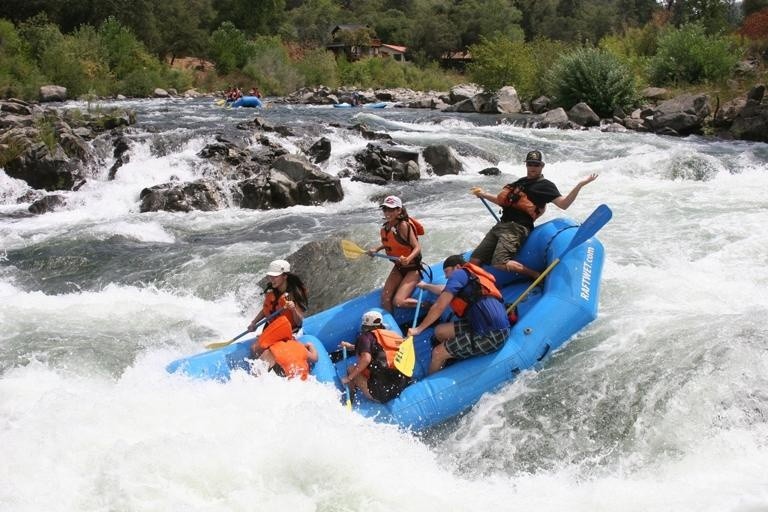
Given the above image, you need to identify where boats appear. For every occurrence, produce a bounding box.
[158,215,607,439]
[333,100,387,111]
[220,95,262,112]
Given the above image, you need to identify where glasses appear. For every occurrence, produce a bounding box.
[382,206,394,212]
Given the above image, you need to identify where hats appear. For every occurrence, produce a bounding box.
[442,255,465,269]
[380,195,402,208]
[523,151,545,166]
[265,260,291,277]
[361,311,383,327]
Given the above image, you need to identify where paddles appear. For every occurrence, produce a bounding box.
[341,239,403,261]
[506,205,612,314]
[394,288,424,377]
[226,101,234,109]
[206,304,287,348]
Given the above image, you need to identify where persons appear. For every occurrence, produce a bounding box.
[341,310,413,406]
[467,151,598,283]
[248,259,309,334]
[369,196,433,316]
[252,316,319,382]
[220,84,264,103]
[406,255,511,377]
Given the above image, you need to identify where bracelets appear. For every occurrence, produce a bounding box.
[347,377,352,381]
